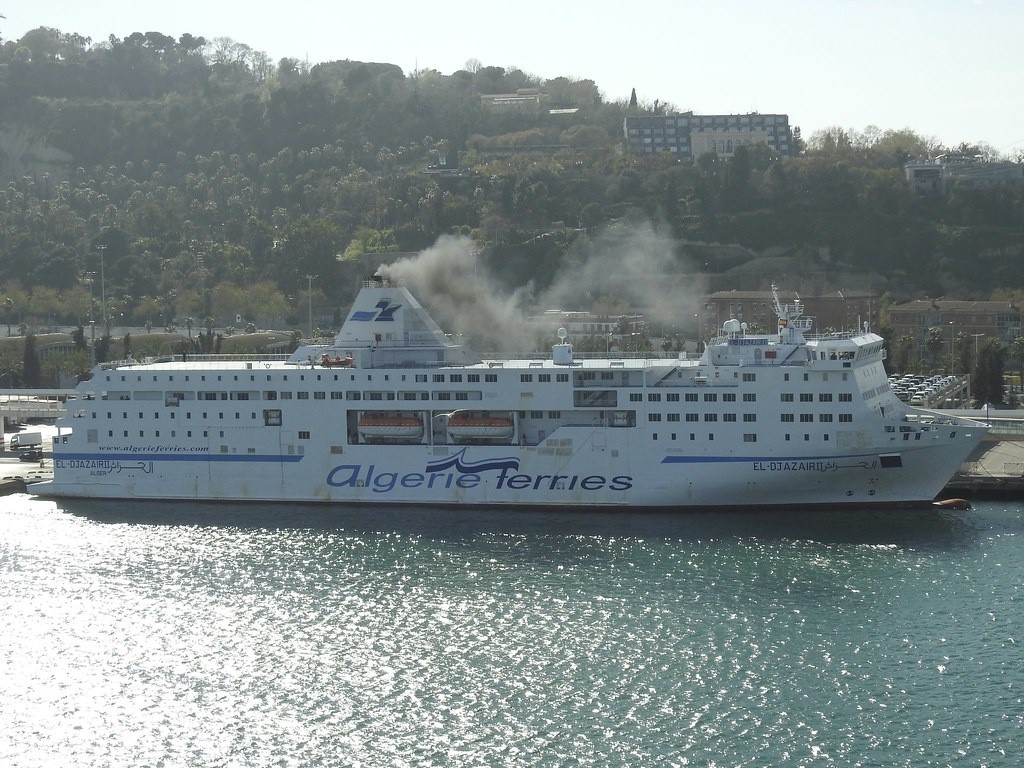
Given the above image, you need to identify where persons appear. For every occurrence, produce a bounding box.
[181,350,187,362]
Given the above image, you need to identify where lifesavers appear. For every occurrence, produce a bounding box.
[265,364,270,368]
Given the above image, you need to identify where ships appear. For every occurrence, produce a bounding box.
[26,276,994,510]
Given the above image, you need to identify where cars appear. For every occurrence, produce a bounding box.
[19,450,43,462]
[889,374,957,406]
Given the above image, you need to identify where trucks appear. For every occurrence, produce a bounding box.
[1001,374,1021,385]
[10,432,42,450]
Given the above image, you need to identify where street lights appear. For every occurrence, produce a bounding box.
[305,273,321,338]
[694,312,702,353]
[603,332,614,357]
[97,243,109,326]
[948,320,957,376]
[969,334,984,376]
[632,332,641,352]
